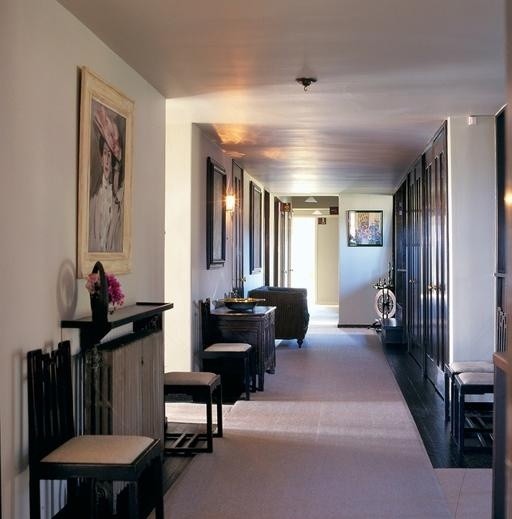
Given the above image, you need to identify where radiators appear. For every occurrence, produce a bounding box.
[97,328,163,515]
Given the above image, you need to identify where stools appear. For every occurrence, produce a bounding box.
[444,362,494,457]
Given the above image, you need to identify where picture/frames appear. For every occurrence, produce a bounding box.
[348,210,383,247]
[76,66,135,280]
[250,181,262,276]
[206,156,226,270]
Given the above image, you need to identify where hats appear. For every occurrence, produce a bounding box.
[93,105,121,161]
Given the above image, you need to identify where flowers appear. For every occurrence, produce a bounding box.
[85,273,125,314]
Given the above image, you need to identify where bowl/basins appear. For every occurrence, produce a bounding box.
[216,297,266,311]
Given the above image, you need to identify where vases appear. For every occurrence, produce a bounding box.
[90,261,109,321]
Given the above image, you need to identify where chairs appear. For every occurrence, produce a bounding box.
[248,285,309,348]
[164,372,222,453]
[27,340,163,519]
[199,298,256,400]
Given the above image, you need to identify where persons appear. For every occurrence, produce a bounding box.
[88,135,125,254]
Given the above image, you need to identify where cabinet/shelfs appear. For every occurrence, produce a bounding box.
[210,306,277,391]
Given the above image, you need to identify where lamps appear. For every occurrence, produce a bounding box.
[304,196,318,203]
